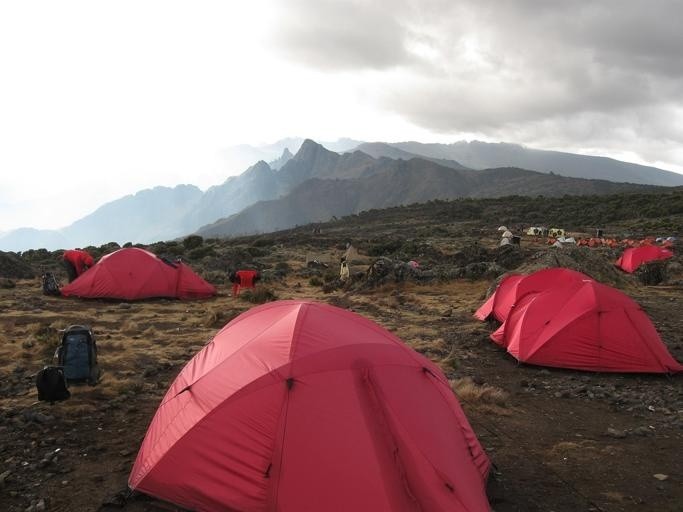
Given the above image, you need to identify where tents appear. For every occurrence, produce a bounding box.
[58,247,216,299]
[477,226,683,374]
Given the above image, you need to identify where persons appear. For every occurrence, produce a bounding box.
[229,270,261,299]
[62,250,93,283]
[126,299,493,512]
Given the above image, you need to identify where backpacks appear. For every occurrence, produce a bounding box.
[36,366,71,401]
[52,325,99,387]
